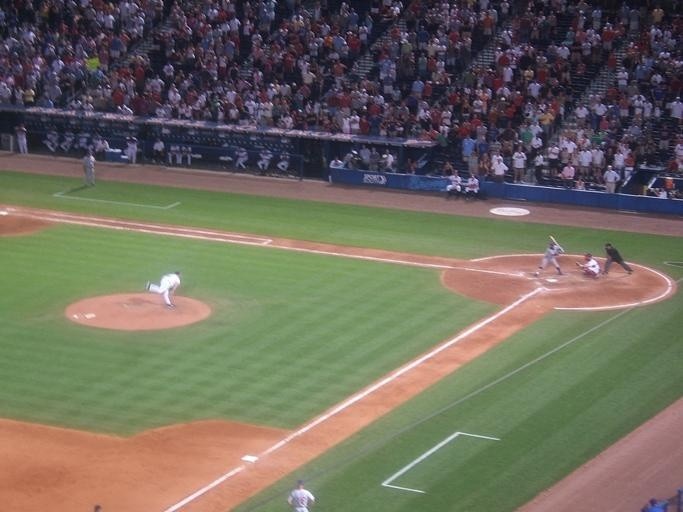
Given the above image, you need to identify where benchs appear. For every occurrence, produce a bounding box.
[0,1,683,200]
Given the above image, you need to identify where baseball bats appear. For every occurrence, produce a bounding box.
[549,235,566,253]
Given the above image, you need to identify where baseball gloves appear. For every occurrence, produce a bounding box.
[575,262,580,266]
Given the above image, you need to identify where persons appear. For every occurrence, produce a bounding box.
[1,1,682,200]
[573,252,600,279]
[532,241,561,276]
[148,268,182,307]
[286,479,315,512]
[640,497,669,512]
[602,242,634,276]
